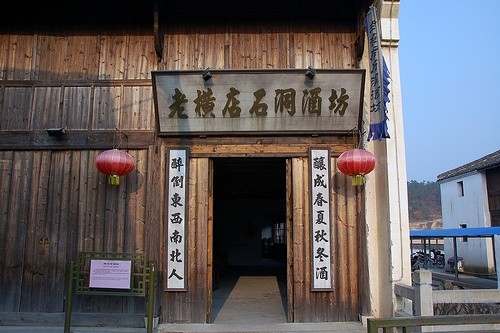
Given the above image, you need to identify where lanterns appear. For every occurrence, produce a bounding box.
[96,149,136,185]
[338,148,376,185]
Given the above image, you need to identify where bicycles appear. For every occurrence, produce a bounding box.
[411,249,445,268]
